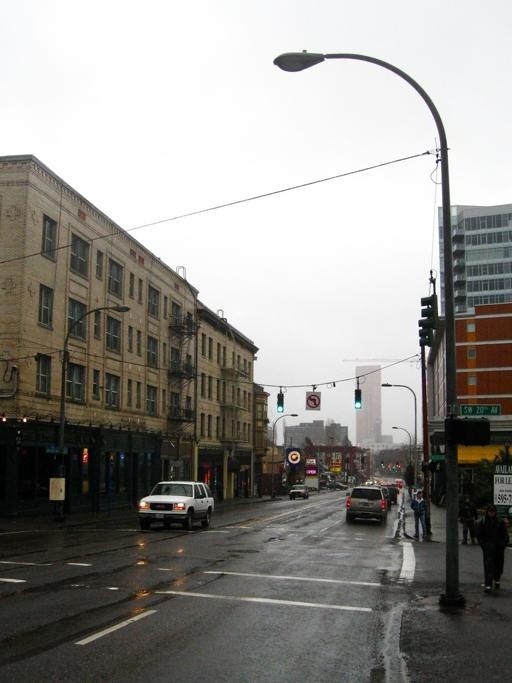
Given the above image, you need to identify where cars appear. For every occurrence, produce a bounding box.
[363,476,405,510]
[289,483,308,499]
[327,481,348,490]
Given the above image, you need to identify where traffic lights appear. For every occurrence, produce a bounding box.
[421,293,439,330]
[417,318,434,347]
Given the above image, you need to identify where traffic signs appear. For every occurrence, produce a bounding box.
[429,454,446,461]
[458,403,502,418]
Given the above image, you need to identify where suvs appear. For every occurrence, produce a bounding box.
[346,485,388,524]
[139,479,216,533]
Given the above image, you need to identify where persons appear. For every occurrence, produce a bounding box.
[411,490,427,537]
[459,501,479,544]
[421,488,433,535]
[476,504,511,592]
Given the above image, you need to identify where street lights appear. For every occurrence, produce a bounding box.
[270,47,464,620]
[277,393,284,413]
[354,389,361,408]
[54,305,130,526]
[271,413,298,498]
[380,383,418,489]
[393,426,412,488]
